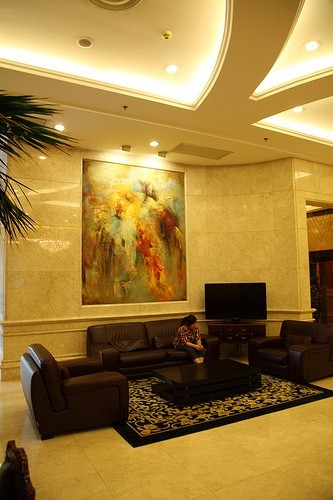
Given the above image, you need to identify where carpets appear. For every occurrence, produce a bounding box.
[111,359,333,448]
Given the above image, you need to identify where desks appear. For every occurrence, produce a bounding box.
[151,358,262,408]
[208,322,267,358]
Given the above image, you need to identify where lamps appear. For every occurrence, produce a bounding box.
[121,145,130,152]
[158,151,166,159]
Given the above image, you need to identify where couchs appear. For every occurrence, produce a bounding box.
[86,318,221,379]
[20,344,129,441]
[248,319,333,382]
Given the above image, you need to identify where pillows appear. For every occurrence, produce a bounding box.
[152,334,172,349]
[111,339,135,353]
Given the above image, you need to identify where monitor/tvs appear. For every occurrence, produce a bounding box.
[204,282,267,324]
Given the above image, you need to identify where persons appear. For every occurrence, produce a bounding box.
[174,315,207,364]
